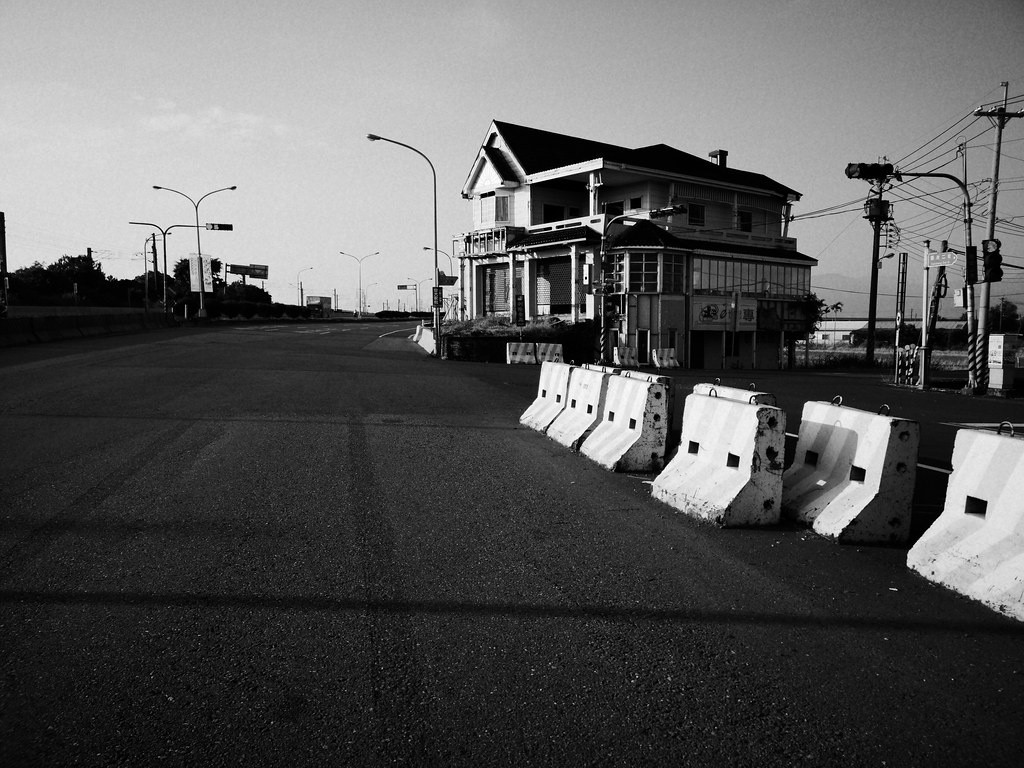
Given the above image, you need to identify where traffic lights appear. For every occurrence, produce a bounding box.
[844,162,894,180]
[982,238,1004,283]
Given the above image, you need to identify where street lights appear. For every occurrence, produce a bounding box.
[367,131,442,356]
[297,266,314,306]
[152,183,237,317]
[407,277,433,312]
[423,246,454,277]
[339,251,379,320]
[143,232,172,315]
